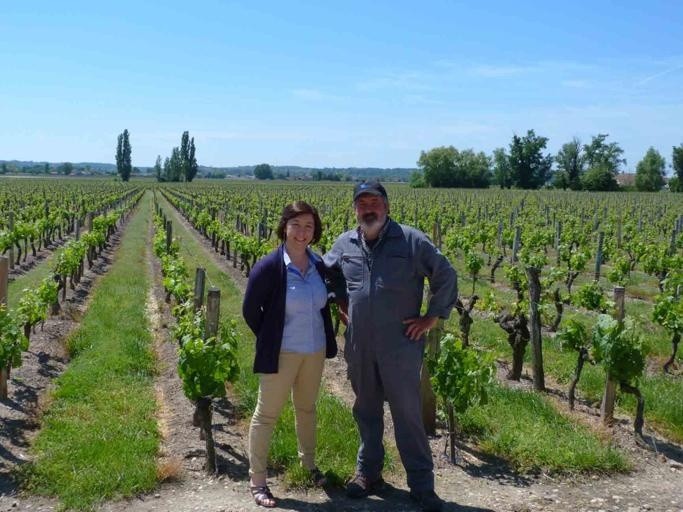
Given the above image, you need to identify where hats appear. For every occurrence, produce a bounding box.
[352,180,387,201]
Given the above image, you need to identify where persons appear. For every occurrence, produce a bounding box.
[239,199,340,507]
[319,180,460,510]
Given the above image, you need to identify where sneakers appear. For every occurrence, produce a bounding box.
[409,487,443,512]
[344,474,384,498]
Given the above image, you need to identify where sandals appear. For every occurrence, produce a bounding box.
[251,486,277,507]
[308,466,328,488]
